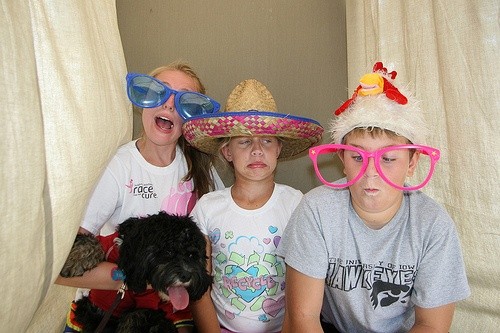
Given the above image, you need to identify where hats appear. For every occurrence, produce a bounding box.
[181,79,324,162]
[333,61,427,144]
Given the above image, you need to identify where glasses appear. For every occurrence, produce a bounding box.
[308,144,441,191]
[126,73,221,121]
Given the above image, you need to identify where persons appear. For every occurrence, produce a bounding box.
[275,61,473,333]
[54,63,226,333]
[182,79,324,333]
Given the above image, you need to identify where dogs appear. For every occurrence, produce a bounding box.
[58,209,216,333]
